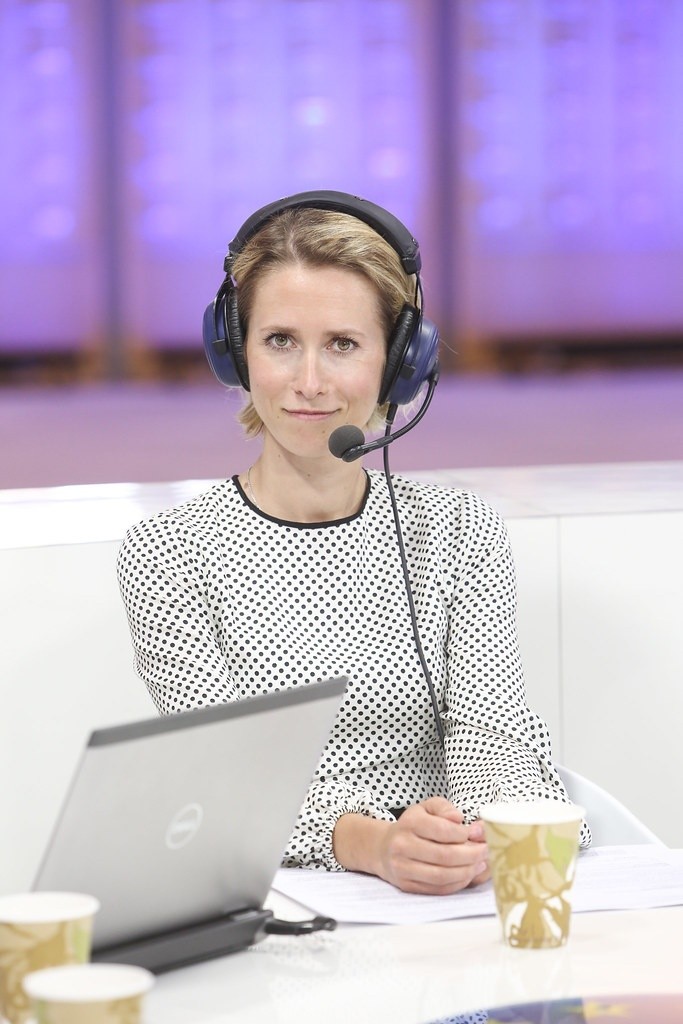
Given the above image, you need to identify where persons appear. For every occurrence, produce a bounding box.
[118,189,592,896]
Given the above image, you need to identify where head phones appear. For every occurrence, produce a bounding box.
[200,190,440,429]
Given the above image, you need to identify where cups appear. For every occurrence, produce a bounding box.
[21,962,156,1024]
[479,800,582,949]
[0,892,101,1024]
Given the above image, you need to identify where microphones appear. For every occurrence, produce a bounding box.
[328,381,437,461]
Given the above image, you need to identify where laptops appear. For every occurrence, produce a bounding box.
[29,676,348,972]
